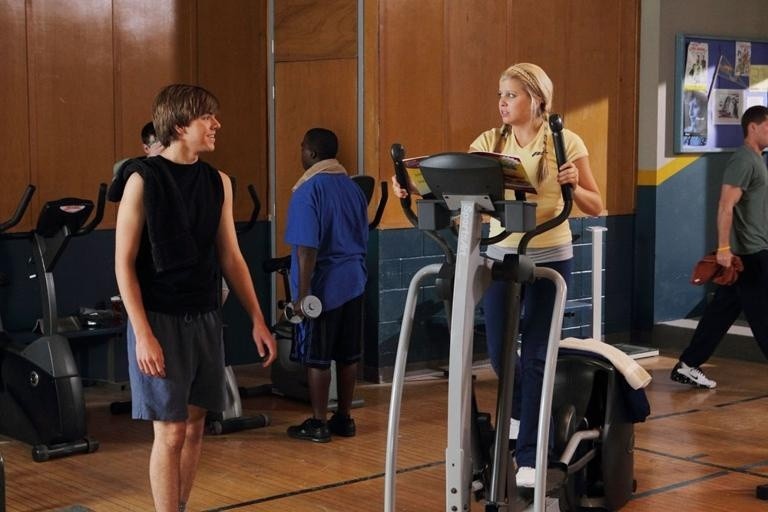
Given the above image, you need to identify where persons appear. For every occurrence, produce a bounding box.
[391,62,604,492]
[689,52,707,84]
[114,82,280,511]
[107,121,167,203]
[282,127,371,443]
[684,88,709,144]
[665,104,768,392]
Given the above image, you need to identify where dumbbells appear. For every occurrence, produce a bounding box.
[283,295,322,325]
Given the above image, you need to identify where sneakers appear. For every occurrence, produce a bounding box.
[329,415,355,436]
[670,360,717,390]
[515,466,536,487]
[288,417,330,443]
[508,419,519,439]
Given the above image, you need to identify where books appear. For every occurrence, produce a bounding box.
[398,152,539,201]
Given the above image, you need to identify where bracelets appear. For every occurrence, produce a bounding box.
[716,245,731,252]
